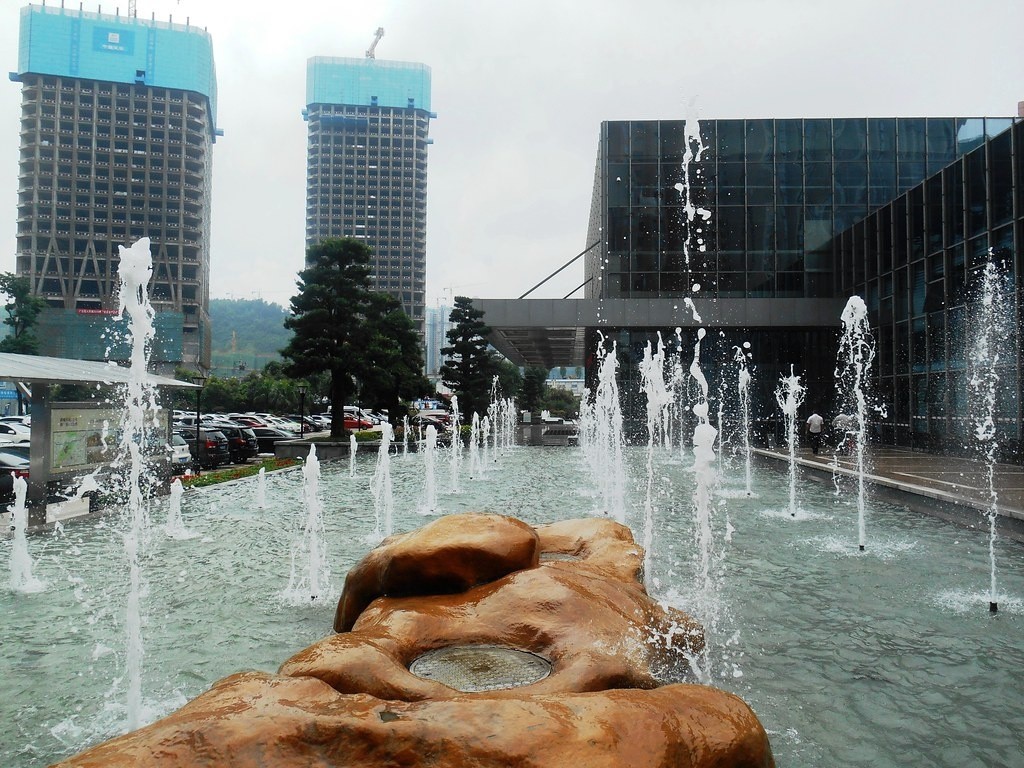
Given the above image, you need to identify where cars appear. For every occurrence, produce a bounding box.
[172,431,191,475]
[253,427,302,453]
[0,414,31,513]
[413,413,465,432]
[173,405,388,434]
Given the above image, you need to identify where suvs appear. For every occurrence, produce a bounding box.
[174,427,229,470]
[199,424,259,465]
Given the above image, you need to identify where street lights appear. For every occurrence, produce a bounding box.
[297,386,309,439]
[191,376,207,477]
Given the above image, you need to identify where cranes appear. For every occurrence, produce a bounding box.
[366,27,385,59]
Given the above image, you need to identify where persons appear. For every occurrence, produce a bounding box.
[831,407,865,457]
[804,408,824,456]
